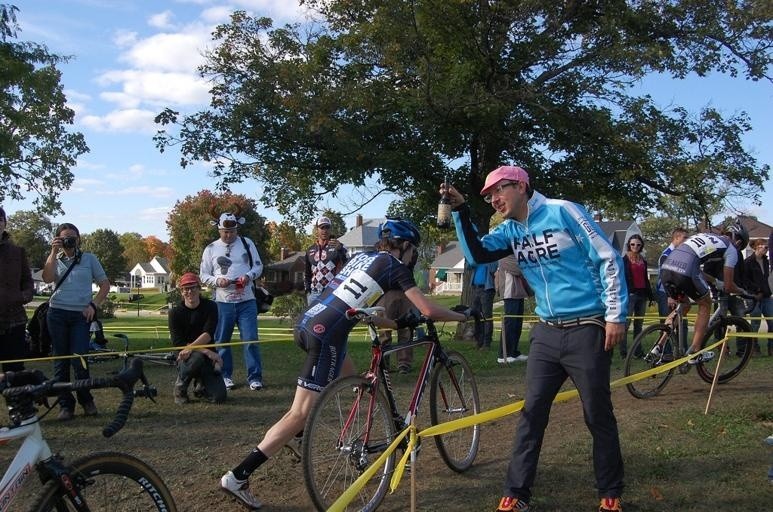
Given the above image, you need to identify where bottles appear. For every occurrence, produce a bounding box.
[437,176,453,235]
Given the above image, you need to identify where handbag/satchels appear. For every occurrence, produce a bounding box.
[252,286,274,315]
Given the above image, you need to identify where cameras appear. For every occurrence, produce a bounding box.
[62,237,76,248]
[230,281,239,284]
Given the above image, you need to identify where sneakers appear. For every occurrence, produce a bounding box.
[222,377,235,389]
[599,497,623,512]
[219,470,263,509]
[192,378,203,398]
[172,383,190,404]
[284,431,306,459]
[493,496,530,512]
[497,356,519,364]
[82,399,98,416]
[516,354,529,361]
[399,364,410,375]
[620,343,773,365]
[56,407,76,419]
[249,381,263,391]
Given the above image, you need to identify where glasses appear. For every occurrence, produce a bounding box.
[629,243,642,247]
[183,285,198,293]
[483,181,517,204]
[758,244,768,249]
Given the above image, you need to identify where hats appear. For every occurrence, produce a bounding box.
[316,216,331,228]
[210,212,246,230]
[179,272,200,287]
[479,165,530,196]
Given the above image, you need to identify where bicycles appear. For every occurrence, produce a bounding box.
[295,299,491,511]
[621,280,762,401]
[92,330,178,375]
[0,353,180,511]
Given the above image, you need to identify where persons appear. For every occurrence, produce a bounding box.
[437,166,629,510]
[301,216,361,391]
[198,212,274,390]
[466,261,497,351]
[42,223,110,419]
[167,273,226,402]
[620,232,656,359]
[220,218,477,508]
[494,253,533,365]
[0,208,35,371]
[376,241,414,374]
[647,221,772,366]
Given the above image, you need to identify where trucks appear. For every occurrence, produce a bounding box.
[127,293,144,302]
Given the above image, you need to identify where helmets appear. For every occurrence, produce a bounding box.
[377,217,421,246]
[726,222,749,250]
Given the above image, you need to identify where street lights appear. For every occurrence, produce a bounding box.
[135,281,140,318]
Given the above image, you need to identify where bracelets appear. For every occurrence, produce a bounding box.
[89,300,97,313]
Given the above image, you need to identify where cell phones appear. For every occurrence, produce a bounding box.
[329,235,336,242]
